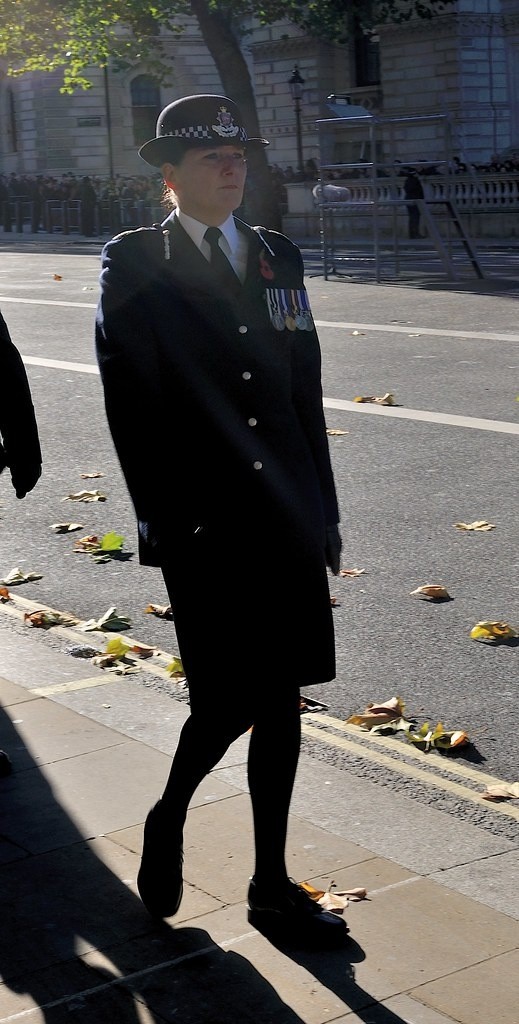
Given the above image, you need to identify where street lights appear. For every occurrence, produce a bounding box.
[65,51,114,184]
[286,64,309,183]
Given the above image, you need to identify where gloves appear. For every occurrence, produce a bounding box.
[323,526,343,576]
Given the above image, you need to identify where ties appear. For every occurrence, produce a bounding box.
[203,227,243,289]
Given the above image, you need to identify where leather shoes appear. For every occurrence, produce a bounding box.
[138,800,185,917]
[248,874,348,937]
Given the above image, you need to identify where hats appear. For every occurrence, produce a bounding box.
[137,94,270,168]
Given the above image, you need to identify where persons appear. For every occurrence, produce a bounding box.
[92,95,352,939]
[0,312,42,500]
[0,155,519,239]
